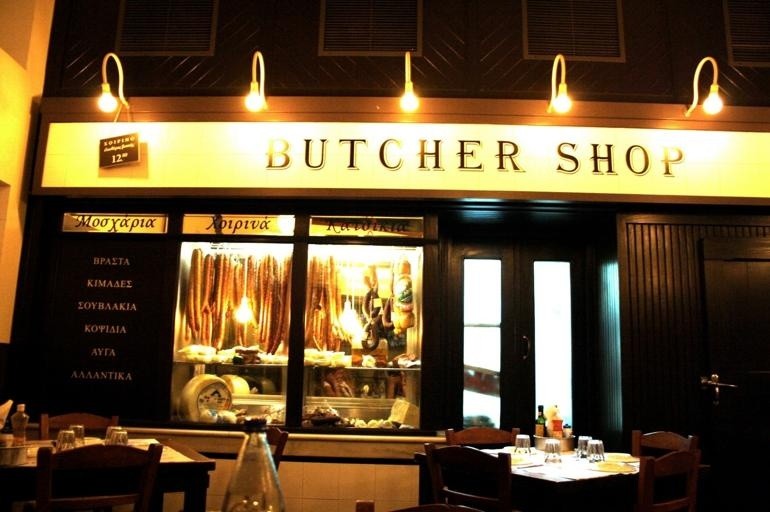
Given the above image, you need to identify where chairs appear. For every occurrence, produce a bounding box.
[353,500,479,512]
[640,436,697,511]
[425,441,515,511]
[631,427,699,488]
[443,426,519,452]
[40,413,120,439]
[222,426,288,512]
[35,444,163,512]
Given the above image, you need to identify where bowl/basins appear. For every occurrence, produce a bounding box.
[533,433,576,453]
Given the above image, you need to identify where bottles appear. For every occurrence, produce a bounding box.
[11,403,30,446]
[534,404,546,437]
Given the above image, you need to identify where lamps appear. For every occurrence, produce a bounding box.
[96,51,130,116]
[684,55,725,118]
[242,49,269,113]
[548,52,573,115]
[400,50,421,113]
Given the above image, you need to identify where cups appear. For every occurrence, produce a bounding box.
[56,424,128,452]
[512,432,605,464]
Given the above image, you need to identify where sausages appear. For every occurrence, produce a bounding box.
[185,245,344,354]
